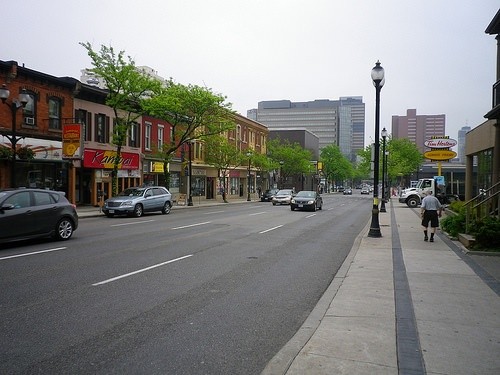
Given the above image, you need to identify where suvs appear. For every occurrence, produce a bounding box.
[103,186,172,218]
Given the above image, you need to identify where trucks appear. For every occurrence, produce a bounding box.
[399,178,465,208]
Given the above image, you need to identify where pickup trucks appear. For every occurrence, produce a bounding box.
[474,189,490,195]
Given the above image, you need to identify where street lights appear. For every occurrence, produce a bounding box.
[186,142,194,206]
[0,83,31,188]
[280,161,284,190]
[370,59,390,238]
[247,151,252,201]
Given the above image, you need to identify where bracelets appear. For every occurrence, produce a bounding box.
[421,213,423,214]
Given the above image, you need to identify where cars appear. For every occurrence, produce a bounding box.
[0,189,79,248]
[361,187,368,195]
[343,188,352,195]
[261,189,279,202]
[339,187,345,192]
[359,183,382,192]
[272,189,296,206]
[290,190,323,212]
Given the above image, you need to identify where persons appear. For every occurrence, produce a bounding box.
[421,189,442,242]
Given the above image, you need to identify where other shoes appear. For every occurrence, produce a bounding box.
[430,238,433,242]
[424,236,428,241]
